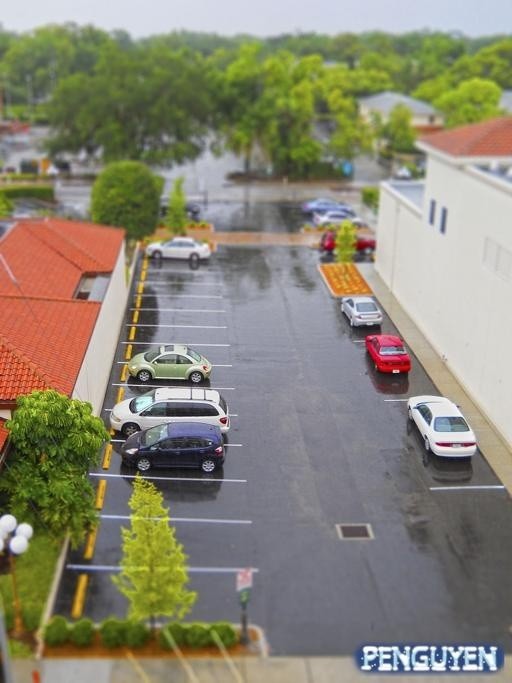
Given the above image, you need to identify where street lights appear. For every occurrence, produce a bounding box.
[0,514,33,642]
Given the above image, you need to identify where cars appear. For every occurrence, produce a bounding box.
[341,297,383,329]
[303,199,376,251]
[366,335,411,375]
[120,422,226,474]
[128,345,212,384]
[109,387,230,435]
[407,395,477,460]
[146,237,211,262]
